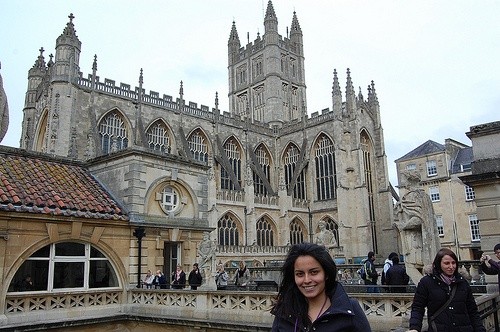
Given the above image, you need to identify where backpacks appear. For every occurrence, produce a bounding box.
[381,262,392,289]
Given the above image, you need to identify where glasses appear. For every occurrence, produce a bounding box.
[494,252,500,255]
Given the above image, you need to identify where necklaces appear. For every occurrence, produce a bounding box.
[307,295,328,320]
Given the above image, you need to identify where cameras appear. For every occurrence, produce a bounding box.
[483,256,487,260]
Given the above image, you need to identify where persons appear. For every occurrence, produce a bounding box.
[144,262,250,291]
[392,169,441,268]
[360,251,409,293]
[19,274,35,292]
[480,243,500,295]
[270,242,372,332]
[314,221,338,262]
[406,248,487,332]
[198,231,215,285]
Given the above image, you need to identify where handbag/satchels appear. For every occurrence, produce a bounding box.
[361,260,371,280]
